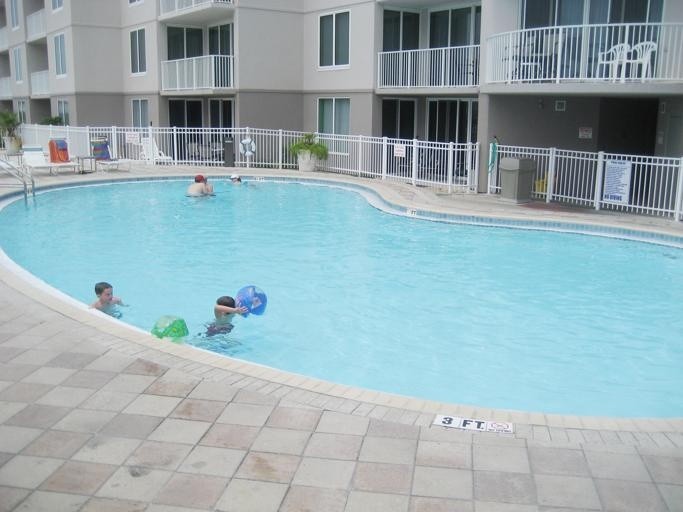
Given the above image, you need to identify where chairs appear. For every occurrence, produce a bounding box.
[139,137,174,170]
[46,136,122,178]
[595,41,658,84]
[502,32,567,80]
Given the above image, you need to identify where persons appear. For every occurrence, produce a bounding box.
[186,175,214,197]
[229,174,242,186]
[202,176,213,192]
[205,294,248,338]
[89,282,129,319]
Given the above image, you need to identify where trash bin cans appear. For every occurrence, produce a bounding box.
[498,157,536,201]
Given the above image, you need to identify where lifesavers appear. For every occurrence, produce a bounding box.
[239,138,255,156]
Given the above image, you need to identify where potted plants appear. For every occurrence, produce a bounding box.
[0,109,26,156]
[288,132,329,172]
